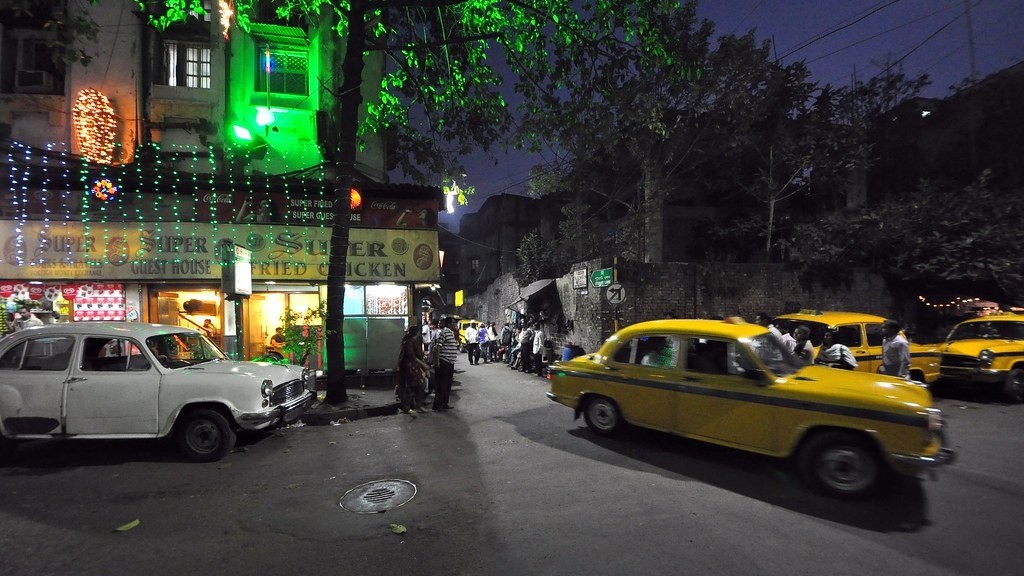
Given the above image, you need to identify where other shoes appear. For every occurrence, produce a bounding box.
[433,405,455,411]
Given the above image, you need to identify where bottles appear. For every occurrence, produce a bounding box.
[58,307,69,315]
[57,300,68,303]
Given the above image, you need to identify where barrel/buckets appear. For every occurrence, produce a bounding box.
[562,344,579,361]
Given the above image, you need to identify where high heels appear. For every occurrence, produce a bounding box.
[417,406,428,412]
[398,407,417,413]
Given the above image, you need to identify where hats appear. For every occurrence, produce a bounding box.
[469,320,475,323]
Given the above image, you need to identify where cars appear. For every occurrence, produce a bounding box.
[768,309,942,384]
[925,311,1024,404]
[545,316,955,505]
[0,322,317,461]
[456,319,485,352]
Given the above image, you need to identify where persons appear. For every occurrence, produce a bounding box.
[814,326,857,371]
[396,313,545,414]
[877,320,911,380]
[641,337,667,368]
[199,319,217,338]
[270,328,286,345]
[6,307,44,333]
[742,311,813,365]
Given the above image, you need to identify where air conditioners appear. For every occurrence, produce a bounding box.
[15,69,52,92]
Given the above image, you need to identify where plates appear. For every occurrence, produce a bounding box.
[413,243,433,270]
[391,237,408,254]
